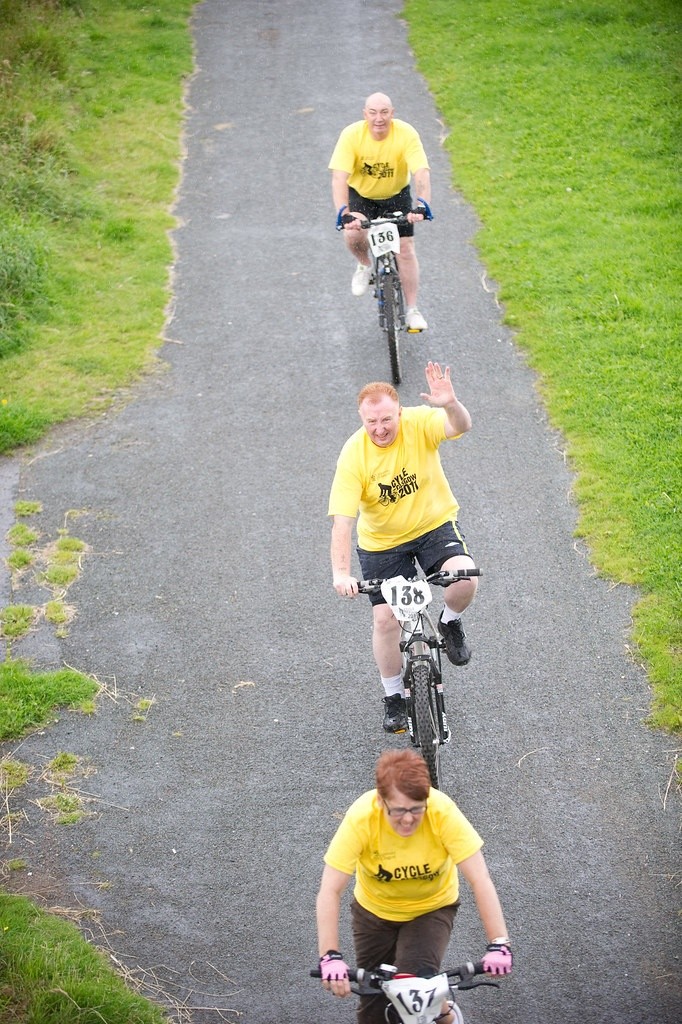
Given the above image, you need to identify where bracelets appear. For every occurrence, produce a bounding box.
[492,937,510,945]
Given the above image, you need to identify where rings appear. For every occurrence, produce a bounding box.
[439,376,443,379]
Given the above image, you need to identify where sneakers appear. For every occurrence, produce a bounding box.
[435,611,471,665]
[381,692,408,732]
[403,305,429,332]
[352,259,373,296]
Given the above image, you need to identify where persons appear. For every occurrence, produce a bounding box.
[328,360,479,735]
[329,92,431,330]
[317,750,514,1024]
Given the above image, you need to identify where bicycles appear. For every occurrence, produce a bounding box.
[339,553,482,793]
[335,199,434,385]
[310,961,500,1024]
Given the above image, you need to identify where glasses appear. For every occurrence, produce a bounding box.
[383,800,428,816]
